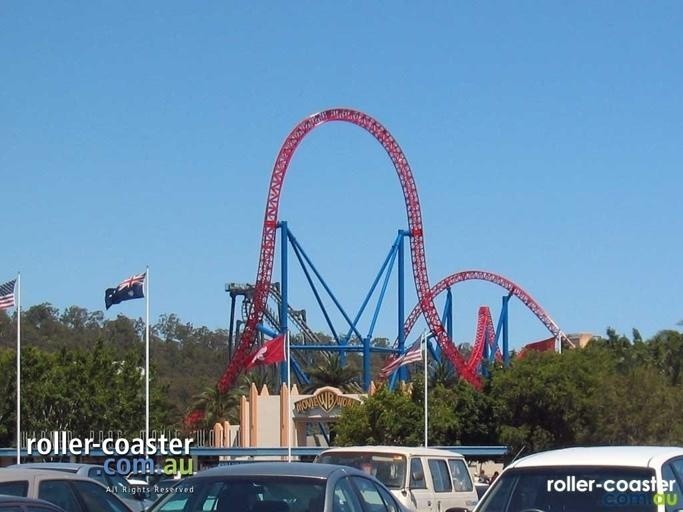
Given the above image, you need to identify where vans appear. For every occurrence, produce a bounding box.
[315,444,479,512]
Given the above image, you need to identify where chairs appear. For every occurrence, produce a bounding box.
[215,482,338,512]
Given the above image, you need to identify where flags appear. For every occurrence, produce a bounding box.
[0,278,16,310]
[243,333,286,368]
[105,272,147,309]
[379,334,423,378]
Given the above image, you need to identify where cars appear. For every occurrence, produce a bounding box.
[473,445,683,512]
